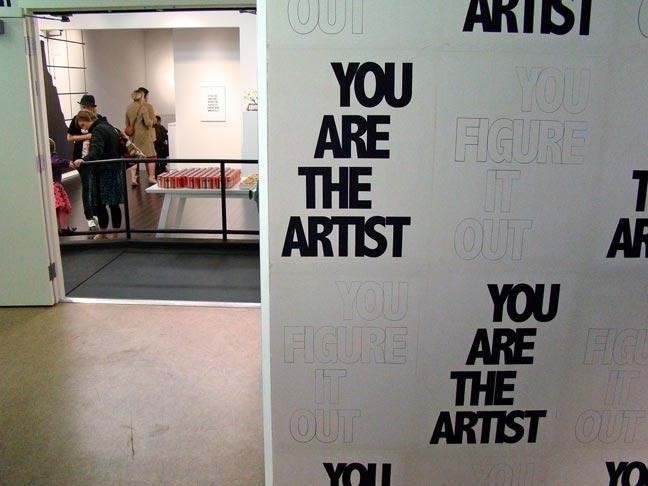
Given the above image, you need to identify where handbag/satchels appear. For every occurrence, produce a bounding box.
[122,141,147,170]
[125,126,135,136]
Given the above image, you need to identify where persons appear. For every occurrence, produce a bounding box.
[132,86,157,183]
[48,137,77,232]
[124,89,157,185]
[66,94,105,227]
[155,114,168,172]
[74,109,122,240]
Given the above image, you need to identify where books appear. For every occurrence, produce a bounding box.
[157,168,240,188]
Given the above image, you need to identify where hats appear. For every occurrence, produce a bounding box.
[77,95,98,108]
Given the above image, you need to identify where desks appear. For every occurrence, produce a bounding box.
[145,176,259,239]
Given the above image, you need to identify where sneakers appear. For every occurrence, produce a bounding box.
[149,175,157,184]
[88,226,122,239]
[132,179,139,186]
[60,226,77,234]
